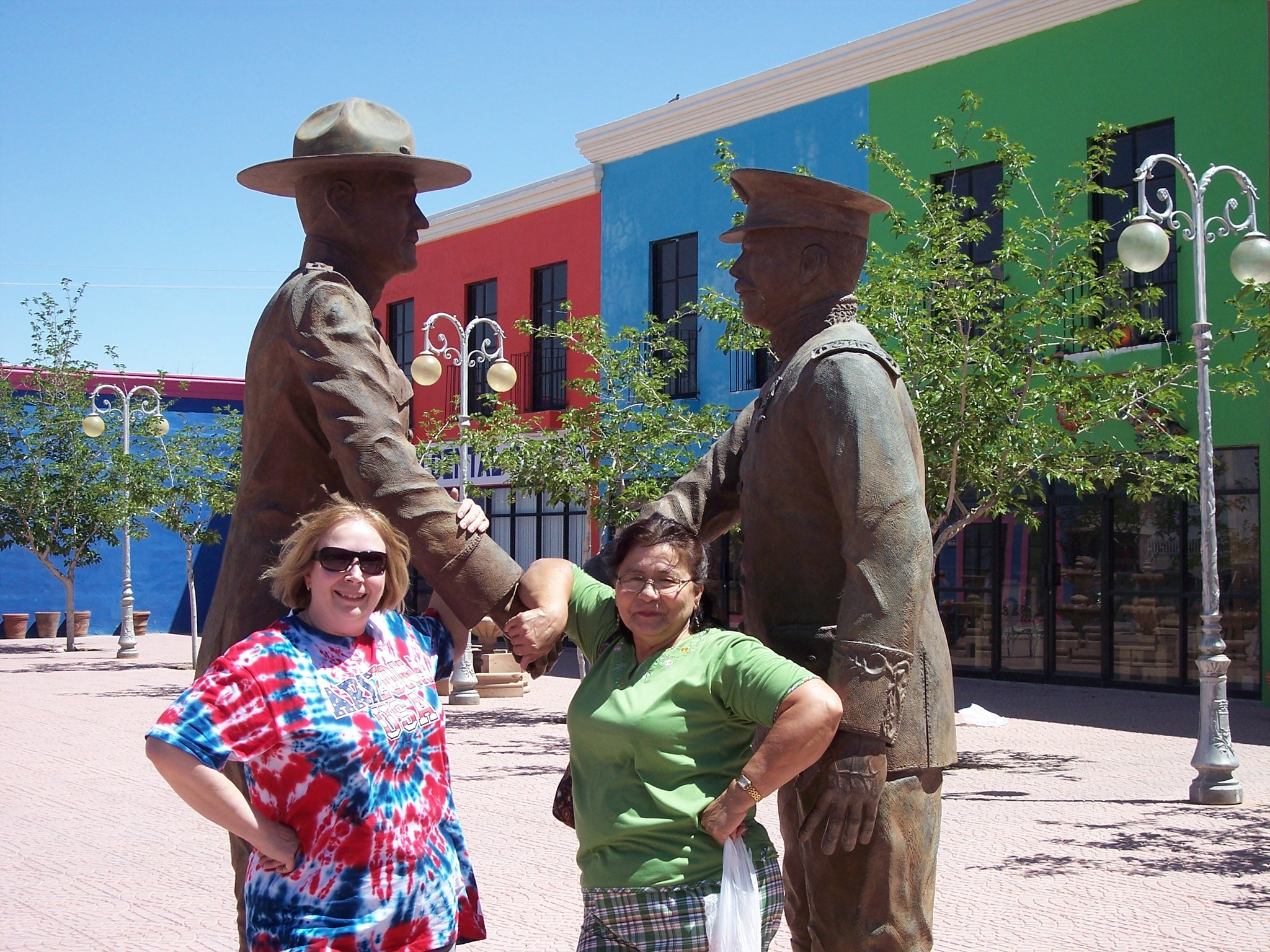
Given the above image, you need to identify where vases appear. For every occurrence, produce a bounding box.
[65,612,91,637]
[35,612,61,638]
[3,614,29,639]
[133,611,151,635]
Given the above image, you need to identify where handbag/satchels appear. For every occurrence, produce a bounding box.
[701,831,761,952]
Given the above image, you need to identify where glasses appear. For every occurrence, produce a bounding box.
[616,573,693,593]
[311,546,387,575]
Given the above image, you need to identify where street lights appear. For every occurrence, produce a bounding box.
[82,382,170,659]
[1118,153,1270,806]
[411,313,517,705]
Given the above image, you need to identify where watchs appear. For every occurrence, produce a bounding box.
[735,771,764,804]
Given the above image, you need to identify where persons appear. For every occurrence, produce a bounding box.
[504,513,846,952]
[142,481,492,952]
[548,166,955,952]
[191,96,561,952]
